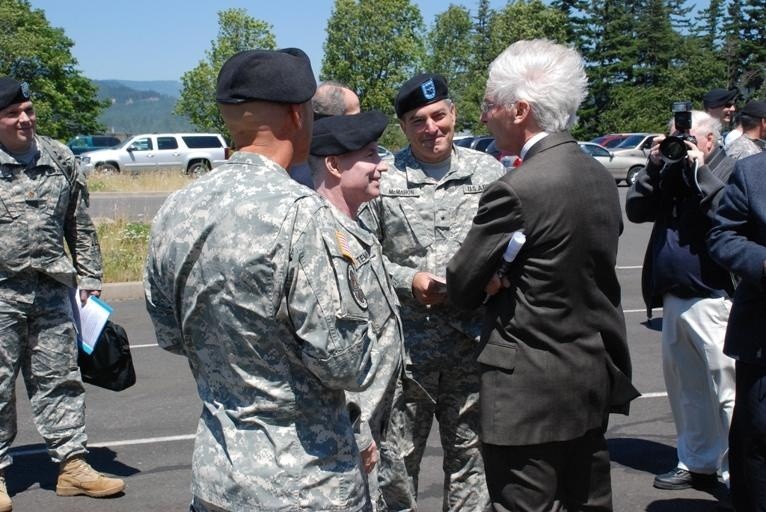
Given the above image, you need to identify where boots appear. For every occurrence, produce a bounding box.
[55,454,125,497]
[0,476,13,512]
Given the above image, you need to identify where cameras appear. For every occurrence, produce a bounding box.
[654,101,698,166]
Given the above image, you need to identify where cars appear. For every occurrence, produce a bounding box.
[590,135,629,149]
[75,134,229,179]
[498,142,647,185]
[469,137,494,151]
[457,138,474,148]
[453,136,473,144]
[65,136,120,156]
[615,134,666,158]
[377,144,392,158]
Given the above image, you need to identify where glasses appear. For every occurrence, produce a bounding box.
[480,101,506,113]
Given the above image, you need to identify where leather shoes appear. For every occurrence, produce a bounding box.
[653,467,718,490]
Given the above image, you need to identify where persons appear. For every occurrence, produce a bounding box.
[142,47,385,512]
[0,75,126,511]
[354,73,507,512]
[710,152,766,512]
[625,108,738,491]
[305,110,437,512]
[287,83,359,191]
[446,36,642,512]
[704,88,766,160]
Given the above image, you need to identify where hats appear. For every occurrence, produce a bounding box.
[743,100,766,118]
[215,48,316,106]
[0,75,30,110]
[310,112,389,157]
[704,88,739,108]
[394,74,448,118]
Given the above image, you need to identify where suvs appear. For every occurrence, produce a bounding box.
[485,140,512,161]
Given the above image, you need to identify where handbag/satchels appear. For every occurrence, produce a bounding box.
[78,320,136,392]
